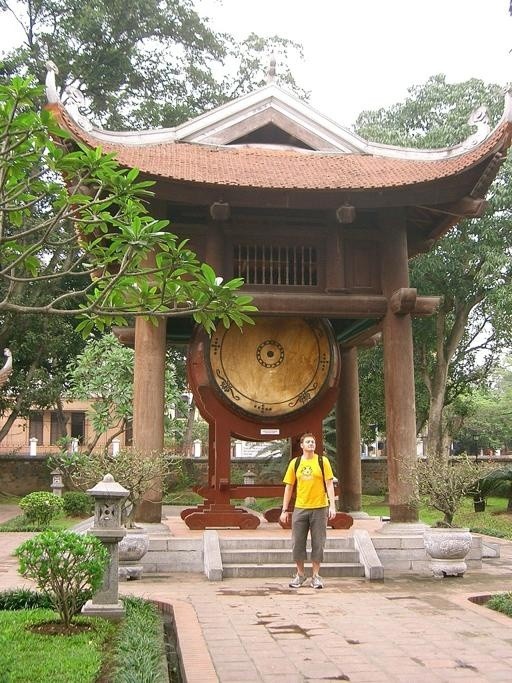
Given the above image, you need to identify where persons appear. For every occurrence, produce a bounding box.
[281,431,338,587]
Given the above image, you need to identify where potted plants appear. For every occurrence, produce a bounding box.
[396,448,507,577]
[50,440,182,582]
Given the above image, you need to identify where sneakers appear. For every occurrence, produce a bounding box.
[289,574,308,588]
[310,575,324,588]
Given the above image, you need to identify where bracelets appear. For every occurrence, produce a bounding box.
[281,508,288,511]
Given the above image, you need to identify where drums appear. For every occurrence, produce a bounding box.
[204,317,335,423]
